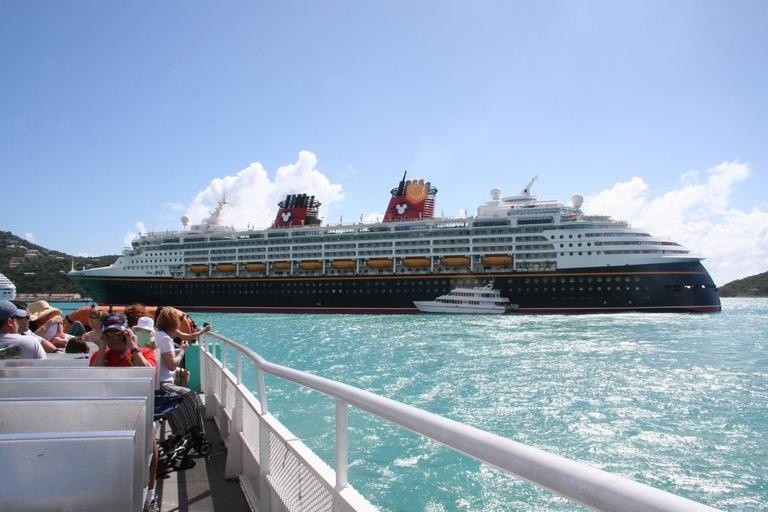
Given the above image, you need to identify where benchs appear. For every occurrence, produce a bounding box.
[0,352,156,512]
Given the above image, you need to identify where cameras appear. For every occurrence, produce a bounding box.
[203,322,211,327]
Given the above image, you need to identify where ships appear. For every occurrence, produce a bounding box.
[66,172,721,315]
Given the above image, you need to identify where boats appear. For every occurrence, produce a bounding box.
[412,280,519,316]
[0,303,719,512]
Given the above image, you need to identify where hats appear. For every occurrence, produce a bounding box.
[101,313,128,335]
[28,299,62,322]
[132,316,157,334]
[0,300,28,321]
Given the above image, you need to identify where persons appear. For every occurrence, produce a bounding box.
[0,300,158,390]
[89,306,212,512]
